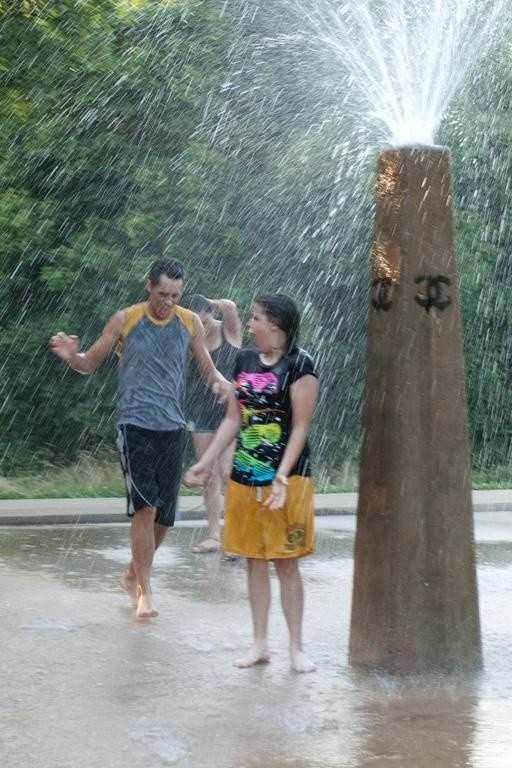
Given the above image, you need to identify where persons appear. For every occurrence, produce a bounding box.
[176,293,242,560]
[50,255,232,622]
[179,291,323,674]
